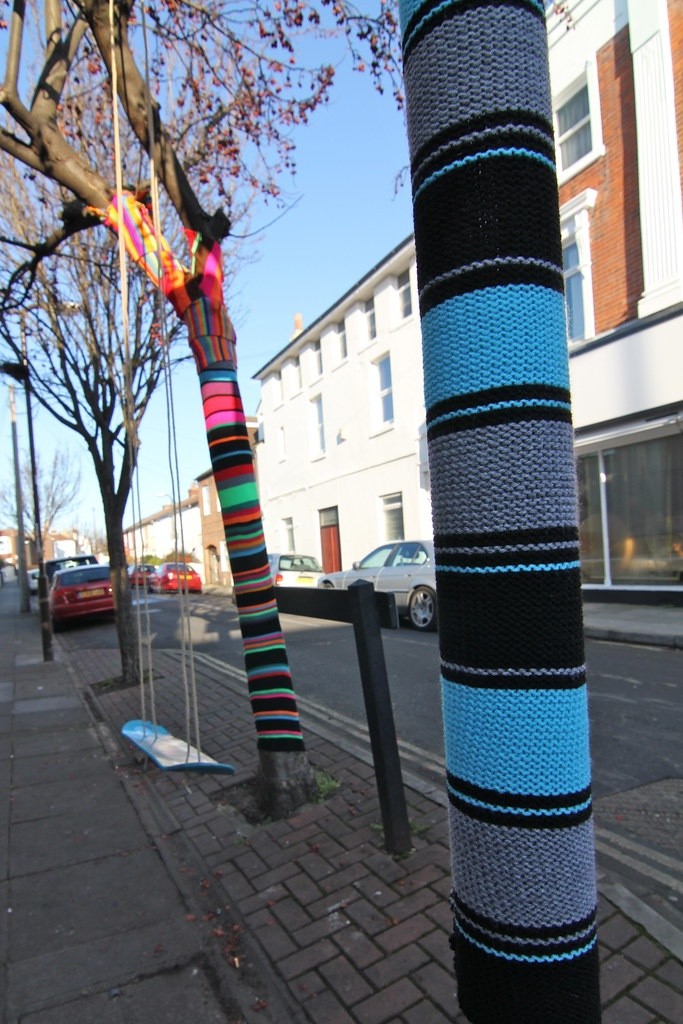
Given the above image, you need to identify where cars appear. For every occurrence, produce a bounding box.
[25,554,116,633]
[315,540,438,631]
[127,561,204,594]
[264,551,326,590]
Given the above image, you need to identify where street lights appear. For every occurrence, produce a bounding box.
[19,299,86,661]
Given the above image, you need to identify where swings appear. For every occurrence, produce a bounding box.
[109,0,236,774]
